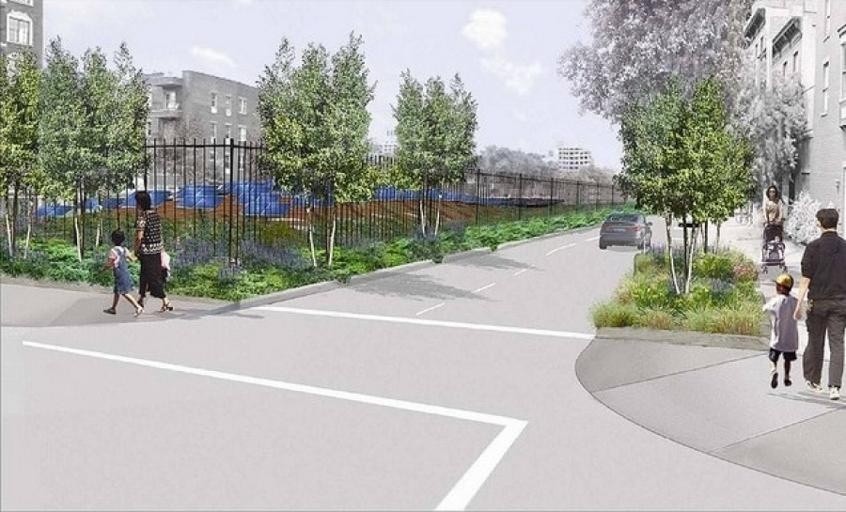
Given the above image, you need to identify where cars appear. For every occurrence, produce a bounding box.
[600,212,653,250]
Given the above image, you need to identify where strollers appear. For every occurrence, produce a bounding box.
[760,221,788,274]
